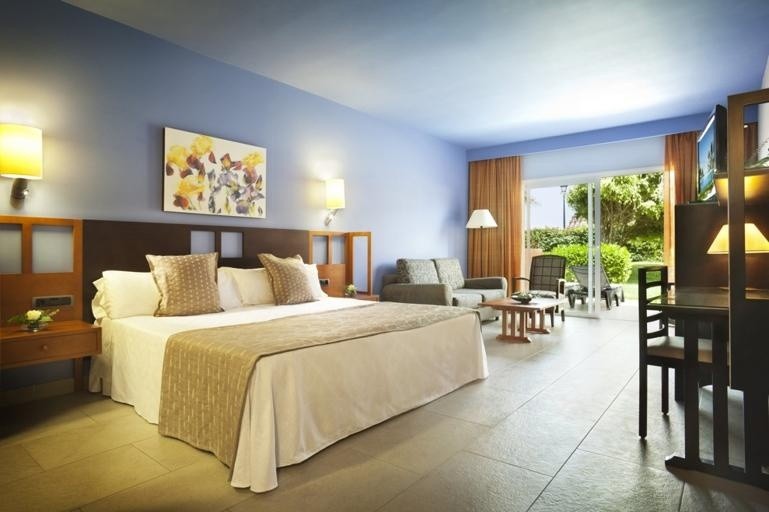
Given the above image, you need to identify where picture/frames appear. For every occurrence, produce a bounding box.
[164,125,267,219]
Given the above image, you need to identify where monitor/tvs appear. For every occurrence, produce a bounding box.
[696,105,728,203]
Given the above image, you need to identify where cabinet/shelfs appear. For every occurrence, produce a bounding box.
[650,89,769,488]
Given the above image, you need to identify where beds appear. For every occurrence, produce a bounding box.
[84,218,489,494]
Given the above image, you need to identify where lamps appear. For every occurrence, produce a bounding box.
[708,222,769,255]
[718,175,768,203]
[30,295,72,330]
[320,178,346,225]
[0,124,44,202]
[467,208,497,277]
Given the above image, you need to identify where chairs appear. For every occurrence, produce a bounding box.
[568,265,625,309]
[638,266,729,439]
[513,254,567,328]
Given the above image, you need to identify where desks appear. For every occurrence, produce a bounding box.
[675,201,769,402]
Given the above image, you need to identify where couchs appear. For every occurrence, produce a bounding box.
[382,257,507,322]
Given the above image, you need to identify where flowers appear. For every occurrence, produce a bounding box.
[15,309,59,331]
[348,284,355,295]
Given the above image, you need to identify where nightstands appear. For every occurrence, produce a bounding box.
[0,215,102,392]
[311,231,379,302]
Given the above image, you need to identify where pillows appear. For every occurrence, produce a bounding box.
[259,253,321,305]
[102,269,241,320]
[230,254,303,303]
[146,252,226,317]
